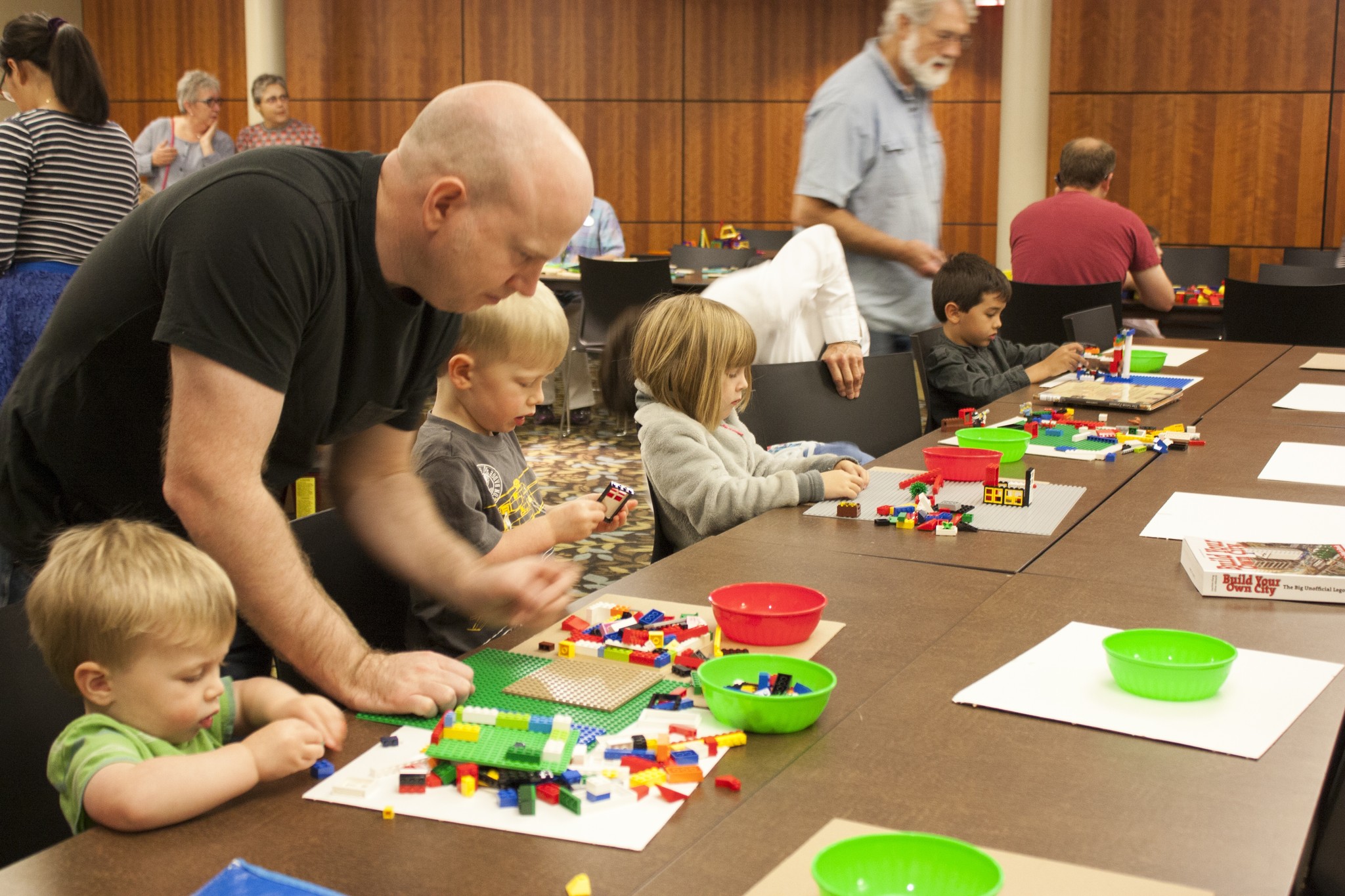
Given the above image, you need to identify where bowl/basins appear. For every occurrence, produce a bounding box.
[698,653,836,733]
[1101,628,1239,702]
[955,427,1032,463]
[812,832,1005,896]
[1121,349,1168,373]
[922,446,1004,481]
[708,581,827,647]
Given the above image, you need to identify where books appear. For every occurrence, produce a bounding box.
[1177,535,1344,606]
[1032,377,1185,414]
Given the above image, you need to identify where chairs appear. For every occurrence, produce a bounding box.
[649,351,922,567]
[1218,278,1345,348]
[669,244,756,272]
[997,281,1124,346]
[559,255,675,438]
[1282,247,1337,266]
[1062,304,1118,355]
[910,327,945,433]
[1257,263,1345,286]
[1159,247,1230,287]
[723,227,794,259]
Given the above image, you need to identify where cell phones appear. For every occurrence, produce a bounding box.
[1054,172,1066,191]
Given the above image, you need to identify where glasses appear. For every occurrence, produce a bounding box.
[196,98,224,108]
[0,67,16,103]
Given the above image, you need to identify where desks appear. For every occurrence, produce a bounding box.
[1122,278,1224,339]
[0,339,1345,896]
[539,262,739,445]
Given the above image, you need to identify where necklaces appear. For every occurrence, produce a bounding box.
[182,117,203,139]
[38,92,58,109]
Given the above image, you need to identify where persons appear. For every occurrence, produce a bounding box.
[0,77,593,863]
[394,276,642,658]
[25,516,352,838]
[1010,137,1177,316]
[0,12,139,402]
[597,221,873,428]
[1118,224,1165,303]
[628,292,869,568]
[786,0,979,371]
[231,71,326,161]
[922,251,1091,439]
[133,70,238,202]
[544,194,628,272]
